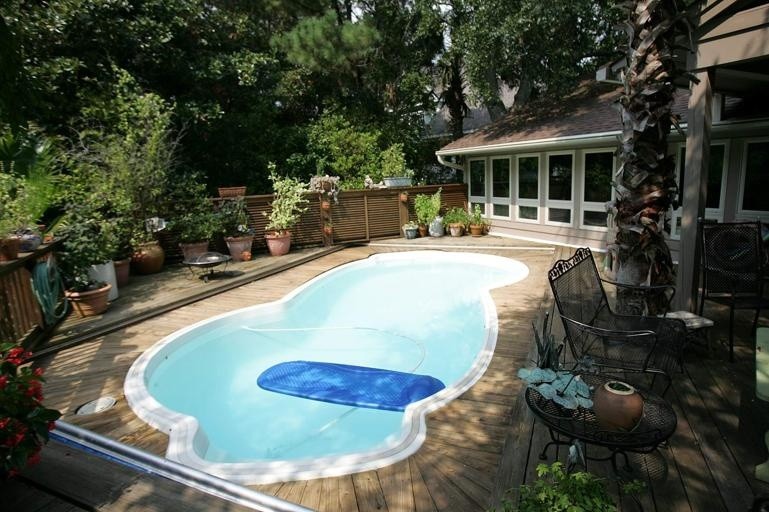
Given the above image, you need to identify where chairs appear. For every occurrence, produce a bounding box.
[547,247,690,399]
[694,219,768,358]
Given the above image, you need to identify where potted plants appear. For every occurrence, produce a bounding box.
[311,175,339,204]
[262,180,311,258]
[174,195,225,262]
[214,197,255,261]
[375,142,412,188]
[400,185,489,240]
[1,108,173,319]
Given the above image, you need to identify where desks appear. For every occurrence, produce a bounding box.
[524,369,678,474]
